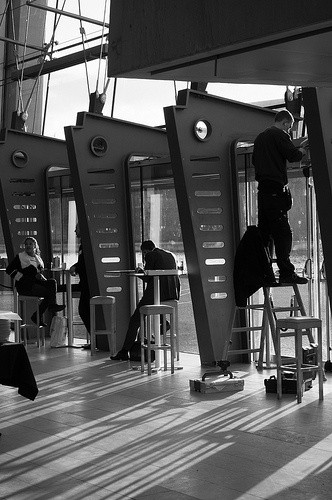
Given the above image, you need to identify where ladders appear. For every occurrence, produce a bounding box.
[221,224,329,382]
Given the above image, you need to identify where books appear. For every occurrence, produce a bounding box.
[0,310,22,344]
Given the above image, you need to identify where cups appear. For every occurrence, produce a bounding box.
[61,263,66,269]
[177,258,183,270]
[139,263,143,268]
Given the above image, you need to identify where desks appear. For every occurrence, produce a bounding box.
[42,266,88,349]
[106,268,184,369]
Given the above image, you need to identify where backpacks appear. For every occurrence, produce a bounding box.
[50,316,68,347]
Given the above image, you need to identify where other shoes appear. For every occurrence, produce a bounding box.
[31,311,47,326]
[81,343,97,349]
[160,321,170,334]
[48,304,66,312]
[110,351,128,360]
[279,272,308,284]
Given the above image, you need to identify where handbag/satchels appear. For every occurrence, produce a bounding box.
[130,340,155,362]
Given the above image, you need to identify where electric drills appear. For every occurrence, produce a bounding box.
[213,359,231,376]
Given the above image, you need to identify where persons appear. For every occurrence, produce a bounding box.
[252,110,308,284]
[70,222,103,350]
[5,237,67,327]
[110,240,181,360]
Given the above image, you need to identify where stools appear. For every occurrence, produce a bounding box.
[90,296,116,356]
[66,291,88,347]
[19,295,45,347]
[134,300,178,375]
[276,317,323,403]
